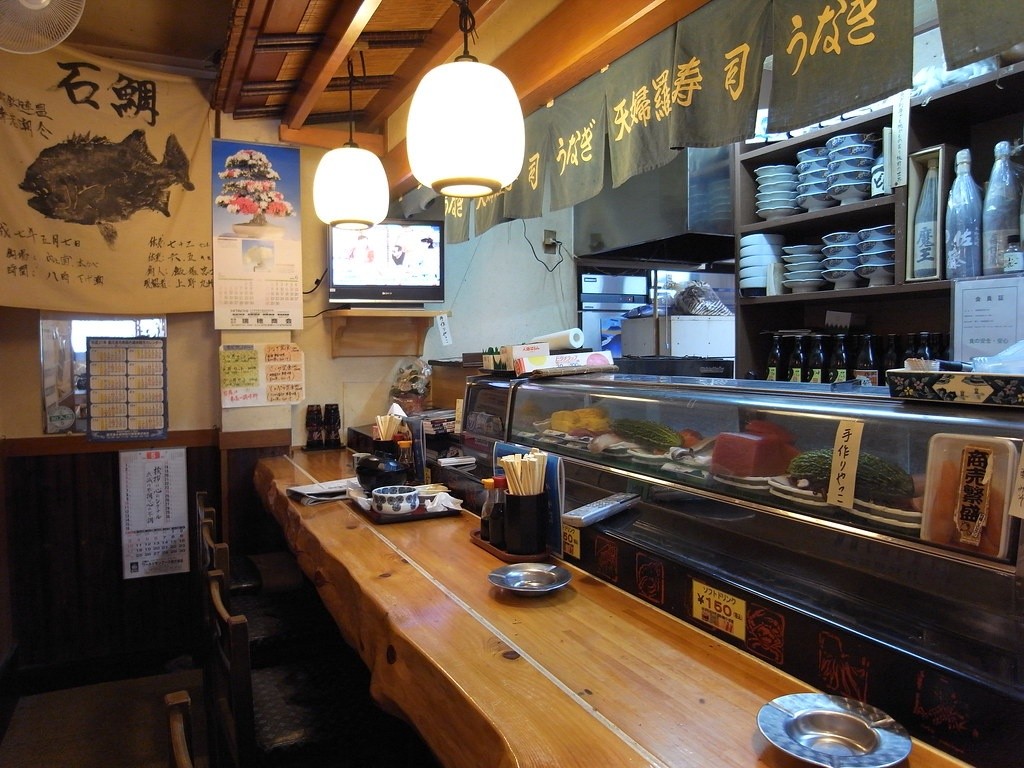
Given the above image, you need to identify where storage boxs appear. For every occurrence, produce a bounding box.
[657,314,736,359]
[620,315,657,357]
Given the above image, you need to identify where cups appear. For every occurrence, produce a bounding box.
[324,404,341,429]
[325,430,340,446]
[505,488,547,553]
[307,430,323,448]
[374,439,394,458]
[306,405,322,430]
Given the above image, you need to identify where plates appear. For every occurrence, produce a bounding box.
[488,563,573,595]
[760,694,912,768]
[544,429,922,531]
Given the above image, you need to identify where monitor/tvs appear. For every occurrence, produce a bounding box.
[327,218,445,311]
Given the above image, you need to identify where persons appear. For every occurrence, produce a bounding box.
[351,236,440,266]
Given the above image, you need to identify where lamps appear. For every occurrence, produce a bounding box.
[312,51,389,232]
[405,1,525,198]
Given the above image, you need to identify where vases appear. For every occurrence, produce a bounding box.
[233,224,286,241]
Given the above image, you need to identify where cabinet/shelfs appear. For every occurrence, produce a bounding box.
[730,54,1024,390]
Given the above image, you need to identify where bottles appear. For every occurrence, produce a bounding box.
[767,333,951,388]
[946,149,983,280]
[398,440,415,481]
[480,479,494,541]
[394,434,405,459]
[1003,234,1024,272]
[913,158,938,278]
[488,477,508,549]
[983,142,1022,276]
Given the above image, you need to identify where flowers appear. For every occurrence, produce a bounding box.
[215,150,296,224]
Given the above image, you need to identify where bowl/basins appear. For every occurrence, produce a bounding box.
[738,133,896,295]
[356,467,407,491]
[417,485,447,503]
[371,486,419,514]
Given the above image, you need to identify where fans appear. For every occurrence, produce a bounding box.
[0,0,88,53]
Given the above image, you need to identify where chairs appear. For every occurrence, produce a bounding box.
[0,491,431,768]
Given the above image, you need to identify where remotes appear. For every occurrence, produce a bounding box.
[561,492,641,527]
[437,456,476,467]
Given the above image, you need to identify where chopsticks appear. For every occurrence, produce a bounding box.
[376,415,402,440]
[500,447,547,495]
